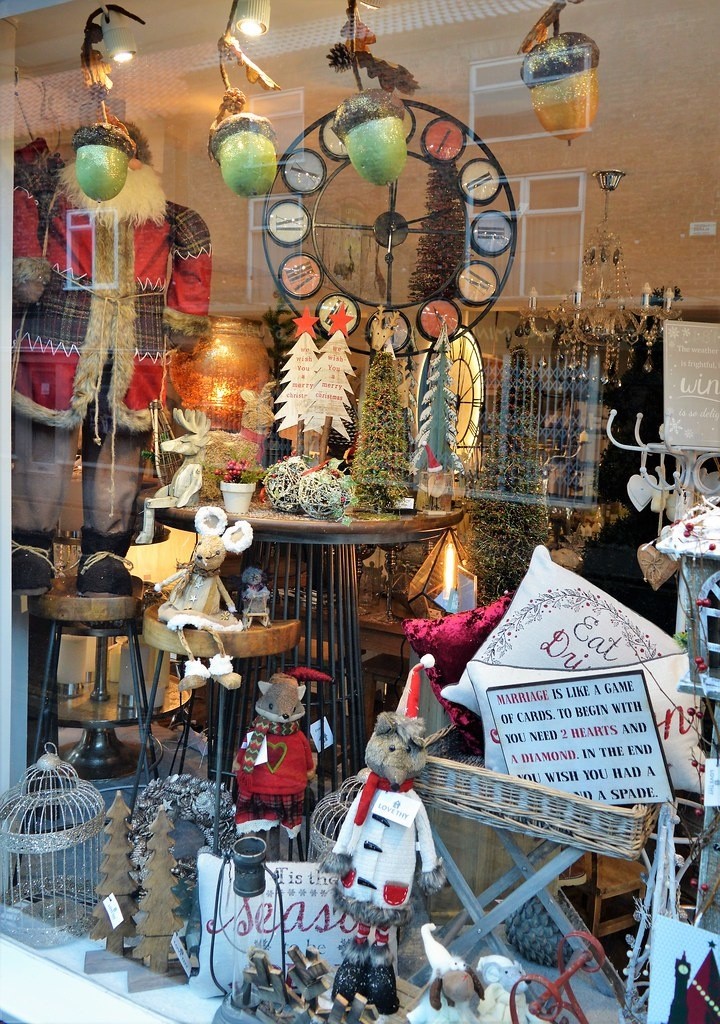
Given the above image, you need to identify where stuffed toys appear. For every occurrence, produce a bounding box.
[476,954,553,1024]
[232,667,335,840]
[199,382,280,502]
[332,654,447,1017]
[142,505,254,689]
[408,923,486,1024]
[239,563,272,630]
[135,408,211,545]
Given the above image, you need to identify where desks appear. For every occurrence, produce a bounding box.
[137,487,465,862]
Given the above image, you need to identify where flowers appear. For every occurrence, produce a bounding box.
[200,447,264,484]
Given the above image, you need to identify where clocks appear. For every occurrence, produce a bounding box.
[262,101,521,357]
[418,326,487,469]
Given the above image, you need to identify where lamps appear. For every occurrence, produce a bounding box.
[360,0,382,10]
[236,0,270,36]
[516,168,681,389]
[101,10,137,61]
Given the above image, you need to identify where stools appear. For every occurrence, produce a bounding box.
[127,601,302,861]
[28,590,159,785]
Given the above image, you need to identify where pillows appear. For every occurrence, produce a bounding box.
[405,544,706,797]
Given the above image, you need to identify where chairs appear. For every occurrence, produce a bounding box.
[564,848,648,938]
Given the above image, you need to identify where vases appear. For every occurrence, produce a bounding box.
[220,481,256,513]
